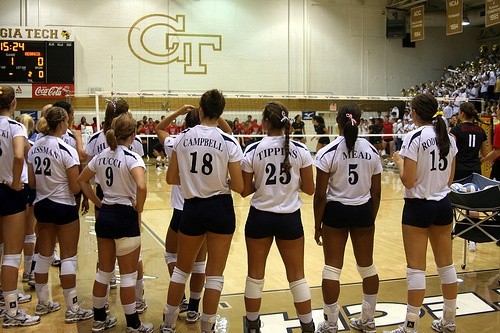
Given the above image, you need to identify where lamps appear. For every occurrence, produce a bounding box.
[463,5,471,26]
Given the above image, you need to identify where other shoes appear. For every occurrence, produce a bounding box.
[154,162,165,168]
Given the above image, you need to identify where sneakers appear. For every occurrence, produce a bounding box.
[134,298,146,314]
[23,272,30,282]
[178,300,190,313]
[186,311,201,323]
[160,323,177,333]
[318,322,338,333]
[17,293,33,304]
[126,322,156,333]
[300,318,315,333]
[110,278,117,289]
[65,303,94,323]
[2,308,41,327]
[35,301,61,316]
[467,240,477,252]
[431,318,456,333]
[91,316,118,333]
[28,274,36,286]
[243,315,262,333]
[51,256,61,266]
[383,325,418,333]
[0,293,5,306]
[348,318,377,333]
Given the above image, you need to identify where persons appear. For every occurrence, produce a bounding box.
[393,49,500,251]
[0,86,154,333]
[391,93,458,333]
[312,102,383,333]
[240,102,315,333]
[310,115,330,153]
[154,89,245,333]
[291,115,306,144]
[359,110,403,169]
[228,115,266,152]
